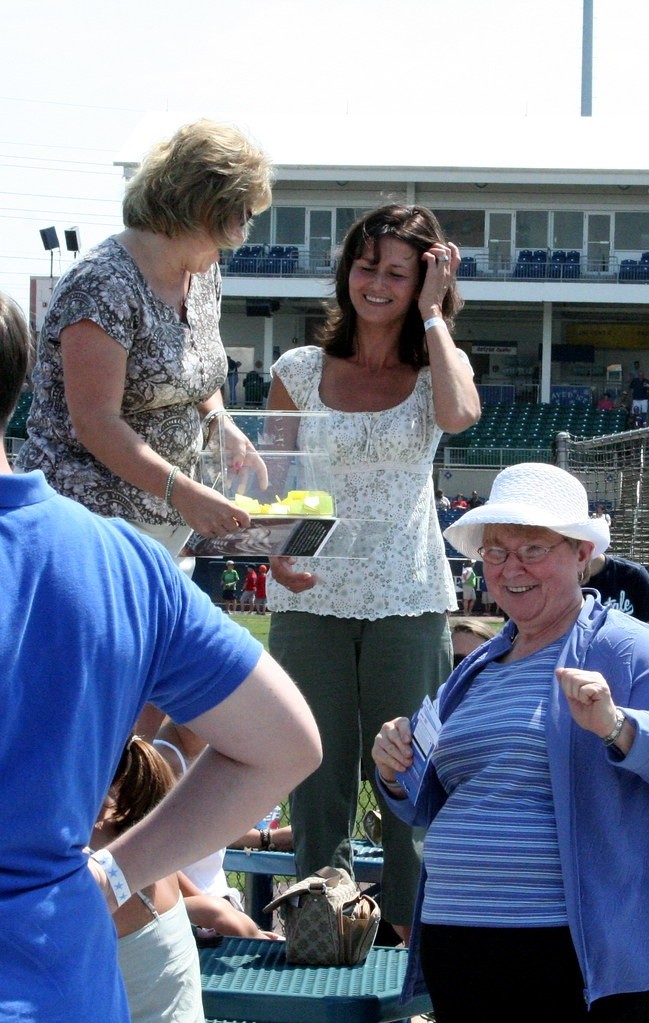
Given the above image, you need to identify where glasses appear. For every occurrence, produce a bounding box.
[479,539,567,565]
[220,197,252,227]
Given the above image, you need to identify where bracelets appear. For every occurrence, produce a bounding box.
[202,408,233,442]
[166,466,181,510]
[261,828,272,850]
[424,317,446,332]
[91,849,133,907]
[379,772,400,788]
[603,709,624,746]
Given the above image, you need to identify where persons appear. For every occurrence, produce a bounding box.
[253,203,479,933]
[582,554,649,624]
[372,463,649,1023]
[222,561,268,615]
[18,118,271,744]
[461,562,500,615]
[597,361,649,411]
[93,724,292,1023]
[225,354,239,406]
[435,489,482,510]
[591,505,611,526]
[0,291,323,1023]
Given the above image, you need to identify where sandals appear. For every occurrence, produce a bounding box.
[190,923,224,948]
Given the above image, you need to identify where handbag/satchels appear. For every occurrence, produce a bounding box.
[263,866,381,966]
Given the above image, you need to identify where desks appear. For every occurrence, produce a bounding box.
[195,839,434,1023]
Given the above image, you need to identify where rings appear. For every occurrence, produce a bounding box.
[438,255,449,261]
[286,585,290,590]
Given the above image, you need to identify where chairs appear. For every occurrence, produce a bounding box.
[460,256,476,278]
[227,244,299,274]
[616,251,649,284]
[228,398,649,607]
[513,250,581,279]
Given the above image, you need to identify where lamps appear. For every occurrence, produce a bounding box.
[336,181,348,186]
[476,182,487,188]
[617,185,630,191]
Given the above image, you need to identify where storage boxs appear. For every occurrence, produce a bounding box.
[196,410,337,523]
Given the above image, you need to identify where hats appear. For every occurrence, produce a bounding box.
[226,560,234,566]
[259,565,267,572]
[442,462,611,563]
[244,564,256,569]
